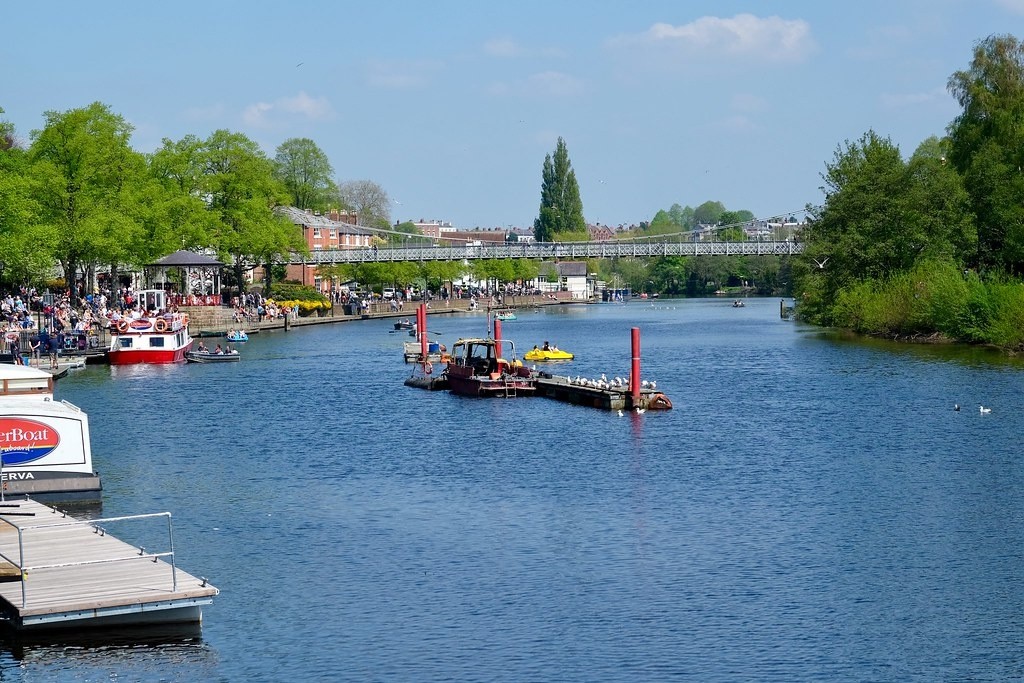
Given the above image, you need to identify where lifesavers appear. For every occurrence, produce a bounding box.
[116,319,130,332]
[425,361,433,375]
[154,320,168,332]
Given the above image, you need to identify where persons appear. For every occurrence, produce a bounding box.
[498,294,501,304]
[232,292,298,320]
[228,328,244,338]
[48,334,58,370]
[29,331,40,368]
[10,332,25,366]
[607,291,621,302]
[735,299,737,304]
[335,290,370,314]
[491,295,498,305]
[534,341,559,353]
[541,293,557,300]
[198,341,231,354]
[390,298,402,311]
[397,318,409,325]
[0,290,179,330]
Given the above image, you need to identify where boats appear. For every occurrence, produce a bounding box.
[106,289,194,366]
[408,329,417,337]
[441,337,539,400]
[183,350,241,363]
[394,322,413,331]
[0,363,104,508]
[227,330,249,342]
[523,347,575,361]
[495,308,517,322]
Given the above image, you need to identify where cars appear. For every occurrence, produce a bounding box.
[384,288,398,299]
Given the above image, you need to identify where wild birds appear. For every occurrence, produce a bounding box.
[617,409,624,417]
[813,258,829,268]
[566,373,657,391]
[954,404,960,411]
[636,408,646,415]
[978,405,991,413]
